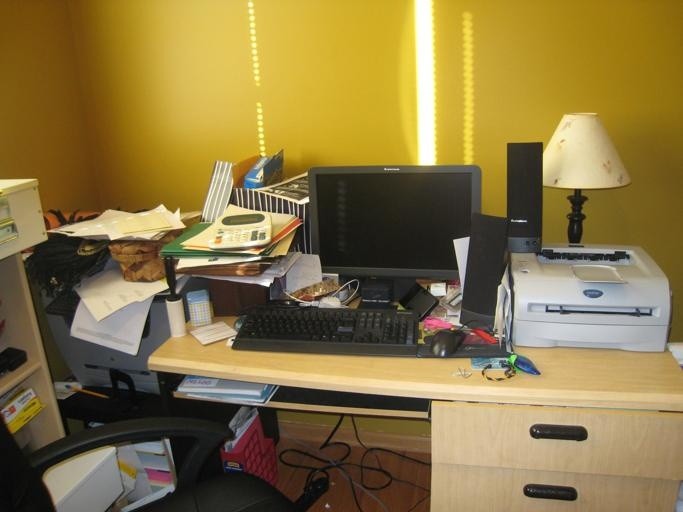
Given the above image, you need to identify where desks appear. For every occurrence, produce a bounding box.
[148,280,683,511]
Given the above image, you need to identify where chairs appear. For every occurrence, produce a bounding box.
[0,412,300,512]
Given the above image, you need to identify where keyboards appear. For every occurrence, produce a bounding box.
[231,305,418,356]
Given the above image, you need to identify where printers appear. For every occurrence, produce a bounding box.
[511,245,671,352]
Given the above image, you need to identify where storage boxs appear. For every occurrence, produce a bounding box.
[218,412,280,487]
[40,287,167,394]
[1,179,49,260]
[180,276,268,317]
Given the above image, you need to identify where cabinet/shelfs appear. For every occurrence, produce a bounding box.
[1,251,65,454]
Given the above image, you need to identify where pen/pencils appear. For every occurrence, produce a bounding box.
[65,385,110,400]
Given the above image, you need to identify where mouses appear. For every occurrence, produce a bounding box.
[431,329,465,357]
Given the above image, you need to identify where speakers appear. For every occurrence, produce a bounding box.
[460,212,508,331]
[508,143,542,252]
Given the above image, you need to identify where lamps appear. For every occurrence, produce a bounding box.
[543,111,632,246]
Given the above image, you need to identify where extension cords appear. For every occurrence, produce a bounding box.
[295,477,329,512]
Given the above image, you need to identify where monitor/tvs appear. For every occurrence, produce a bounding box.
[308,165,481,309]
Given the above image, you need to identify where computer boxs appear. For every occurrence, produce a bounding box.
[169,400,280,487]
